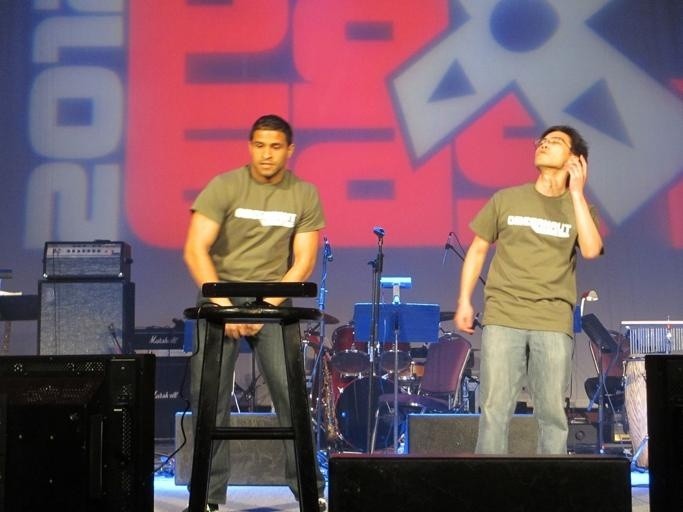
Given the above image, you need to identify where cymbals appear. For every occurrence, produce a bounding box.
[440,311,454,320]
[320,313,339,324]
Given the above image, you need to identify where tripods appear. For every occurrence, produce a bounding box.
[294,300,343,456]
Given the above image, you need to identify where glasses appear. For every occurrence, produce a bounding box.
[535,137,570,146]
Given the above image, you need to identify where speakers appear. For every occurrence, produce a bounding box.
[0,354,156,512]
[405,414,538,456]
[174,411,287,487]
[644,354,683,512]
[328,457,633,512]
[38,279,134,355]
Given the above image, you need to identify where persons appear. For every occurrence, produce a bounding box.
[179,118,330,512]
[453,126,604,456]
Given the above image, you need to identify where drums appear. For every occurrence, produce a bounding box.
[333,325,369,372]
[334,376,409,451]
[379,342,411,373]
[624,355,648,468]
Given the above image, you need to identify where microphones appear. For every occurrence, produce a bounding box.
[440,232,453,266]
[323,235,336,263]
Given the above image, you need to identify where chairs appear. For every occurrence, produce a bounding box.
[365,333,471,456]
[588,329,632,448]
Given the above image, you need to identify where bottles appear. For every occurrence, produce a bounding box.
[397,443,405,454]
